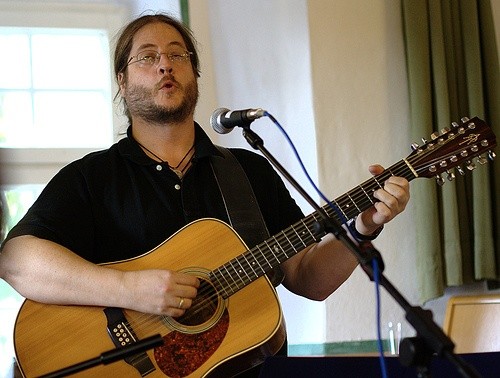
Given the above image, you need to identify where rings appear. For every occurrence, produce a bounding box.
[177,297,184,309]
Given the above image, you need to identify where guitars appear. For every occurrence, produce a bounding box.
[12,111,498,378]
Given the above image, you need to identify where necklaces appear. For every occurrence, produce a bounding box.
[136,139,195,179]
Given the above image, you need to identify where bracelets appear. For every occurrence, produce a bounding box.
[349,213,384,240]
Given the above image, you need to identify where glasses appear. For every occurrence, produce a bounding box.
[119,48,193,72]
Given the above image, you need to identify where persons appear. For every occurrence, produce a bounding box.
[0,7,411,378]
